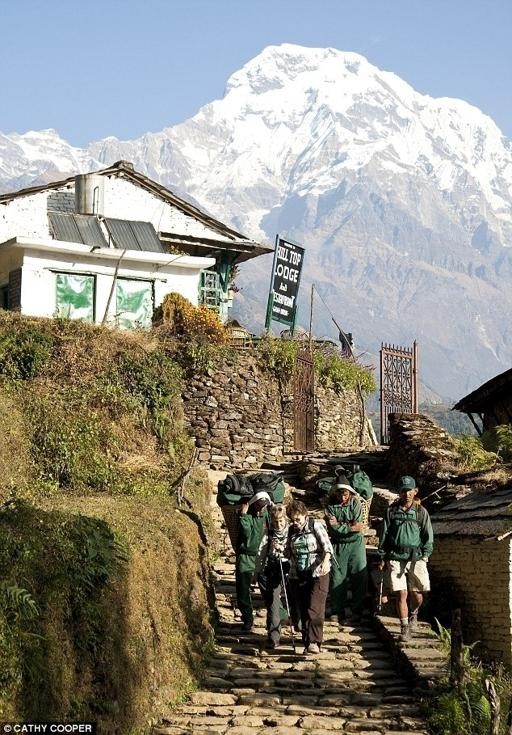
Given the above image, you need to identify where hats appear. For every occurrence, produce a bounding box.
[399,476,416,489]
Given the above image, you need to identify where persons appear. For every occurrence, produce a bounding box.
[233,491,276,630]
[323,475,368,626]
[375,473,435,643]
[282,500,334,656]
[250,502,291,651]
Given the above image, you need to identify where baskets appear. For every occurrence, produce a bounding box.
[221,505,241,552]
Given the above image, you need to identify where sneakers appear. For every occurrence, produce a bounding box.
[331,611,345,622]
[269,638,279,646]
[352,614,362,621]
[303,643,319,656]
[399,612,419,641]
[242,622,252,630]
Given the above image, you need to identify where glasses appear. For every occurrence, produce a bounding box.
[291,518,300,522]
